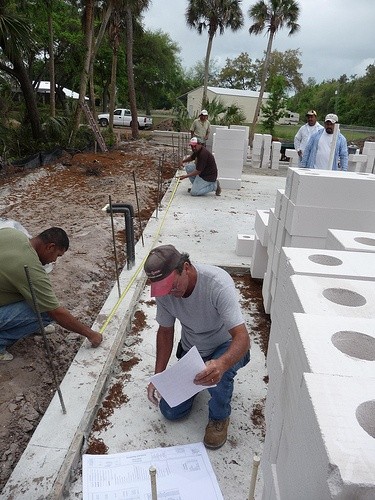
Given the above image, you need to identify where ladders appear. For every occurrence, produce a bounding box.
[81,102,108,153]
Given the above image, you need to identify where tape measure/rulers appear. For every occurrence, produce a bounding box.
[99,177,180,334]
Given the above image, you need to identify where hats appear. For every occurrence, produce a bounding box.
[324,113,339,123]
[189,136,202,146]
[306,110,317,116]
[200,110,208,115]
[143,244,181,297]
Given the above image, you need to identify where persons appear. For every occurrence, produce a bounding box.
[176,137,221,197]
[143,244,251,448]
[294,109,325,161]
[298,113,348,171]
[190,110,211,148]
[0,227,102,360]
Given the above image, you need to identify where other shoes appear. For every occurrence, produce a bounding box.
[0,351,13,361]
[216,180,222,195]
[203,416,230,448]
[36,323,56,333]
[188,188,191,191]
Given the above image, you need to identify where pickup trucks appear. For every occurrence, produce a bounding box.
[98,108,152,130]
